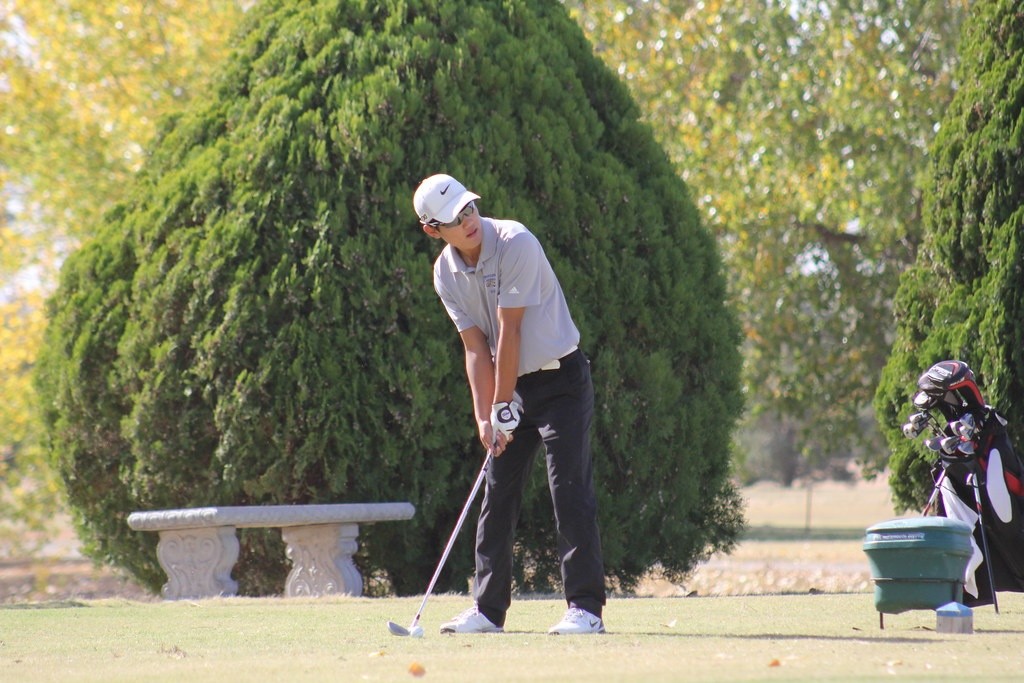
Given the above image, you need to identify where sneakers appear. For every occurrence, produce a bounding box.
[548,606,605,636]
[439,601,505,633]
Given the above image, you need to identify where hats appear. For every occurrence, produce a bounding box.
[413,173,481,223]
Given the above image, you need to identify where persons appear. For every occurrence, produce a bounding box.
[413,174,607,633]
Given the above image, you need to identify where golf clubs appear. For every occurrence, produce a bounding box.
[902,411,979,456]
[386,439,500,637]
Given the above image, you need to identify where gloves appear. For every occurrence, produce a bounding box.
[489,400,522,444]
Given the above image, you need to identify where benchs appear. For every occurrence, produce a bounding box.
[125,502,413,601]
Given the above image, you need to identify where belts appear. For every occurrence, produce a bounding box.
[539,344,579,370]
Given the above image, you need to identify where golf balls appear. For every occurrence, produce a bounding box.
[410,626,423,638]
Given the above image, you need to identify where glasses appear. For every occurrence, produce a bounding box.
[420,200,477,228]
